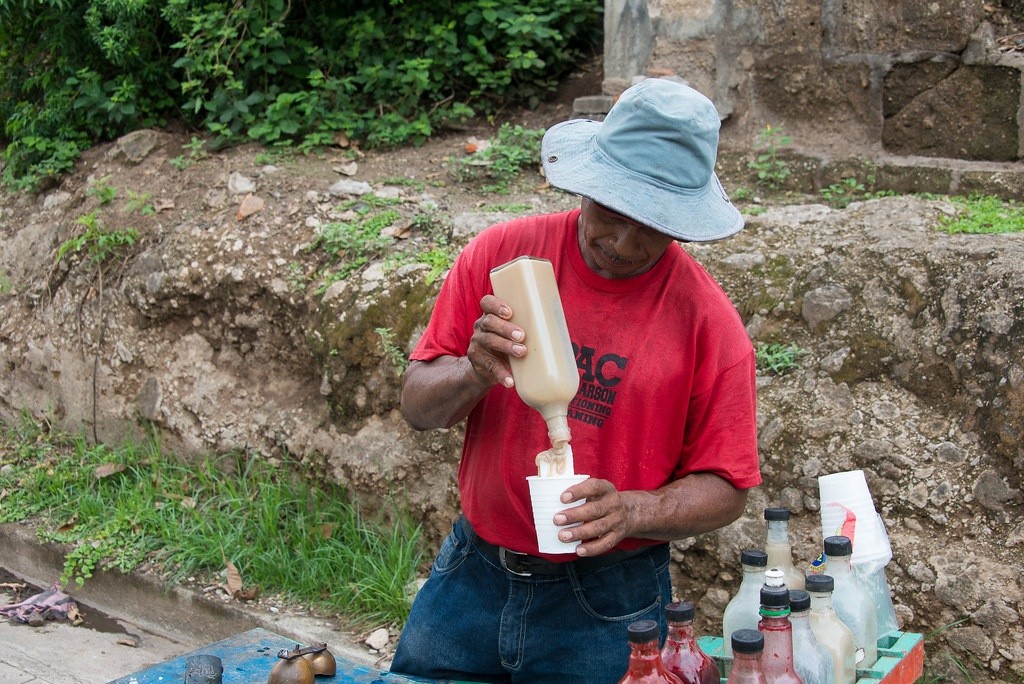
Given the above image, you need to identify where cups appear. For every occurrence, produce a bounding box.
[526,476,589,554]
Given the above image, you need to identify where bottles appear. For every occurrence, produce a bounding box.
[764,507,806,590]
[821,536,879,669]
[660,602,720,684]
[788,590,830,684]
[617,620,684,684]
[723,549,768,656]
[726,629,768,684]
[804,574,857,684]
[490,254,582,447]
[758,567,803,684]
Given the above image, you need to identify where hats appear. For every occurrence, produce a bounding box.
[540,78,745,240]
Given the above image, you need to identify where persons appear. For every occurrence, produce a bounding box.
[389,77,762,684]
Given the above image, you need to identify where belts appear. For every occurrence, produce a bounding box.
[460,515,656,576]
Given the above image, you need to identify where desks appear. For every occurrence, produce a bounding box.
[103,625,457,684]
